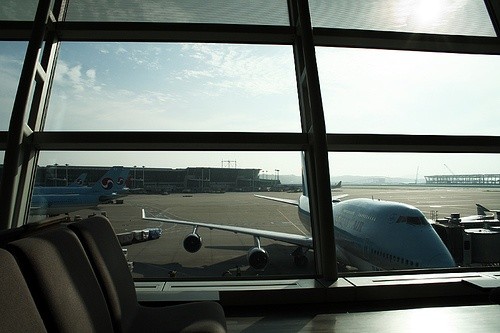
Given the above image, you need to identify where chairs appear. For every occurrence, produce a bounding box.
[0,248,49,333]
[9,228,115,333]
[68,217,226,332]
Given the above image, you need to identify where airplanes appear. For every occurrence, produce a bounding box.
[29,165,143,217]
[141,151,494,272]
